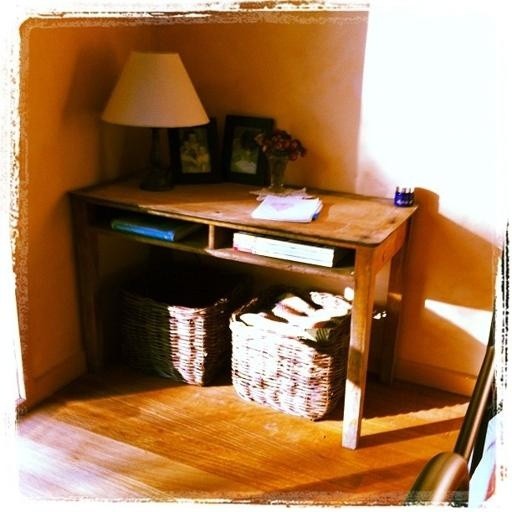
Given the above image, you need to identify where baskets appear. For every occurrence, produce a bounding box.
[119,261,255,387]
[228,284,389,422]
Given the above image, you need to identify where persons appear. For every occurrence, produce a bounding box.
[180,130,202,173]
[184,140,201,160]
[194,144,213,173]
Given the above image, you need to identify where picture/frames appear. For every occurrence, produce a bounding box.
[166,111,275,188]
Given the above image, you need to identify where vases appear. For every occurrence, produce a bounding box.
[267,153,289,189]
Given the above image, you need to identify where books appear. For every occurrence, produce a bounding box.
[111,213,199,242]
[248,193,324,223]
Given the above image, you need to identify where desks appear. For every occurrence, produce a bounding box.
[63,175,419,453]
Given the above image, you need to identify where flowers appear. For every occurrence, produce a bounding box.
[254,128,307,164]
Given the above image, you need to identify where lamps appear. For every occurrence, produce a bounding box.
[101,49,211,192]
